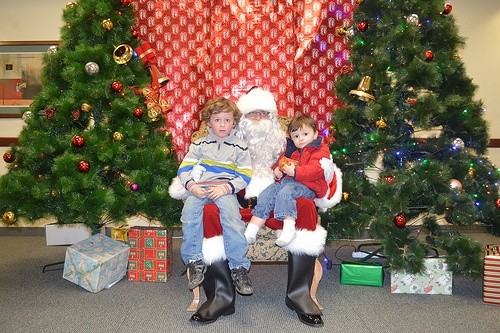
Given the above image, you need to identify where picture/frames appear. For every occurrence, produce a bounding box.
[0,41,61,119]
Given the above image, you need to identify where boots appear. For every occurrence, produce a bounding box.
[276,225,327,328]
[188,236,236,325]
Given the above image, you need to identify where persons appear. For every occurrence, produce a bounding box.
[177,96,254,295]
[244,113,331,247]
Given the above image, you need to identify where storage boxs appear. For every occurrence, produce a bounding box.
[390,257,453,295]
[481,244,500,305]
[45,222,92,246]
[105,226,128,241]
[62,233,131,293]
[339,260,384,287]
[128,226,173,283]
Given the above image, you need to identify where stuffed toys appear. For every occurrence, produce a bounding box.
[168,86,343,327]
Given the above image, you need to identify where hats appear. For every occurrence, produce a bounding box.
[235,86,277,115]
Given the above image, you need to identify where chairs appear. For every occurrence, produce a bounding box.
[186,116,324,311]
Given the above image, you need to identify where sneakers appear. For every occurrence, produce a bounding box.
[231,266,254,294]
[188,259,207,289]
